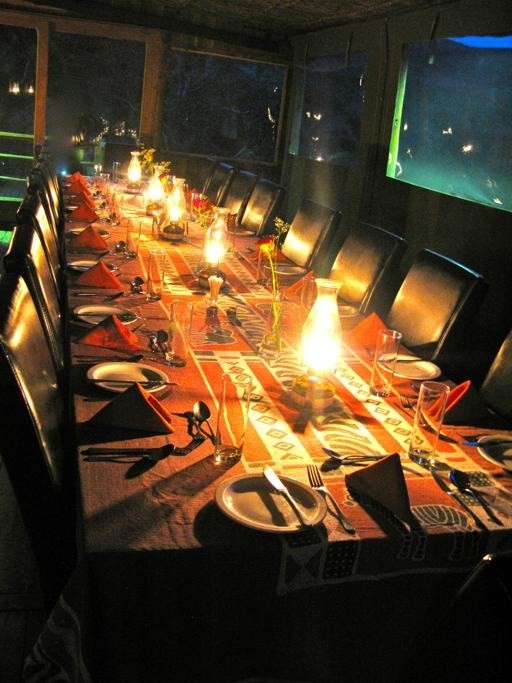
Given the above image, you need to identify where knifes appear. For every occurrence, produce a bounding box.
[87,378,178,388]
[428,467,489,531]
[263,464,311,526]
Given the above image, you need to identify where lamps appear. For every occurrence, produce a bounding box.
[124,151,142,194]
[159,178,185,240]
[144,165,168,216]
[291,278,342,410]
[201,205,231,285]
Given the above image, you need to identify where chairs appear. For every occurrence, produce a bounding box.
[328,220,408,313]
[477,334,511,418]
[240,179,287,236]
[185,154,259,225]
[384,248,483,361]
[2,155,67,383]
[280,197,342,270]
[2,266,206,676]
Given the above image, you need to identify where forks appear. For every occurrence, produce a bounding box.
[69,265,120,272]
[305,465,352,532]
[87,436,205,456]
[77,353,144,364]
[77,311,147,322]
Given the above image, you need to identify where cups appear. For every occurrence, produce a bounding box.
[107,190,123,226]
[165,300,193,368]
[126,218,142,259]
[113,162,121,183]
[167,175,175,192]
[93,165,109,201]
[213,372,250,464]
[408,382,450,467]
[257,242,275,288]
[302,276,317,324]
[145,250,166,302]
[369,329,401,396]
[190,193,202,224]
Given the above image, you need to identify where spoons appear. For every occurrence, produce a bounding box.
[87,444,175,462]
[133,276,146,295]
[448,468,504,526]
[116,240,129,256]
[321,448,401,462]
[194,401,219,445]
[155,329,171,358]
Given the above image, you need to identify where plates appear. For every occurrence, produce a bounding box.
[63,175,102,219]
[214,473,327,534]
[88,361,169,395]
[478,434,509,469]
[228,227,255,238]
[265,261,307,278]
[379,351,441,381]
[68,226,112,241]
[68,260,117,275]
[337,305,360,318]
[74,305,139,326]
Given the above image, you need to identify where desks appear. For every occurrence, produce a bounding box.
[60,174,511,656]
